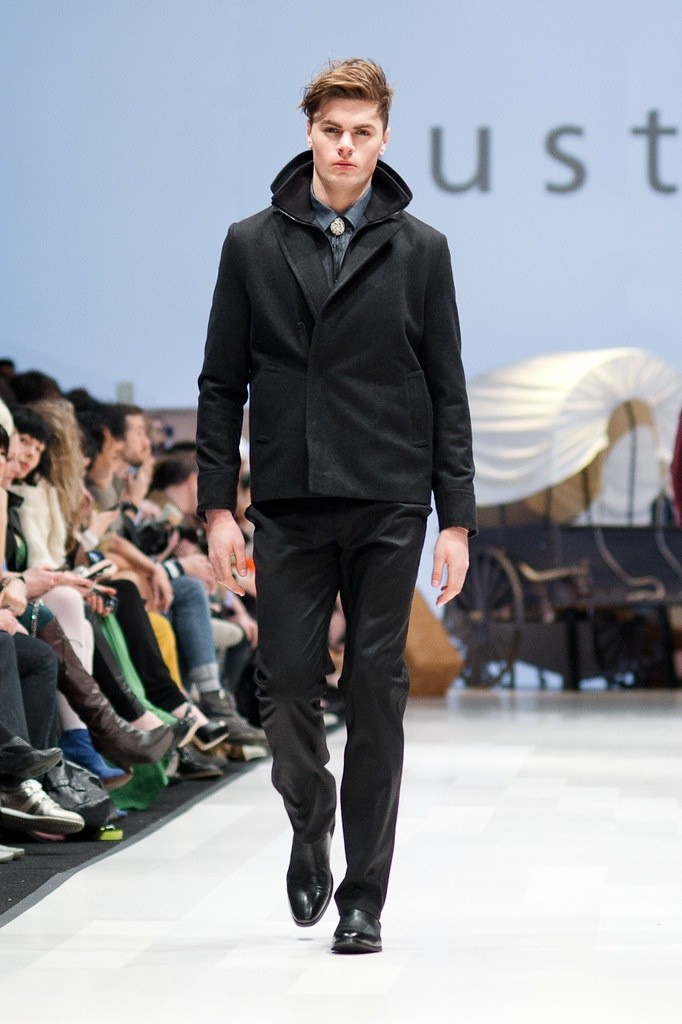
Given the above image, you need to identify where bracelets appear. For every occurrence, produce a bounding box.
[457,526,469,532]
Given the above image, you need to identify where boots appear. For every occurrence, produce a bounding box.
[36,618,174,781]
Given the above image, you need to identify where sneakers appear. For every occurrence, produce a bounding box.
[0,780,85,835]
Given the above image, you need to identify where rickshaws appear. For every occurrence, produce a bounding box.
[441,344,680,689]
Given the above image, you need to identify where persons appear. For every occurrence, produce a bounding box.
[196,57,477,954]
[0,358,267,862]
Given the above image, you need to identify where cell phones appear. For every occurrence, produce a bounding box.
[55,564,69,572]
[89,587,119,612]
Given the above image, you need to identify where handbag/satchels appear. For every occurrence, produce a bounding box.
[38,763,112,836]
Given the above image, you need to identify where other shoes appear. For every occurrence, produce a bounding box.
[2,737,61,785]
[170,683,346,781]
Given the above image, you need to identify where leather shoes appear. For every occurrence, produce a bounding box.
[286,812,336,929]
[332,912,382,958]
